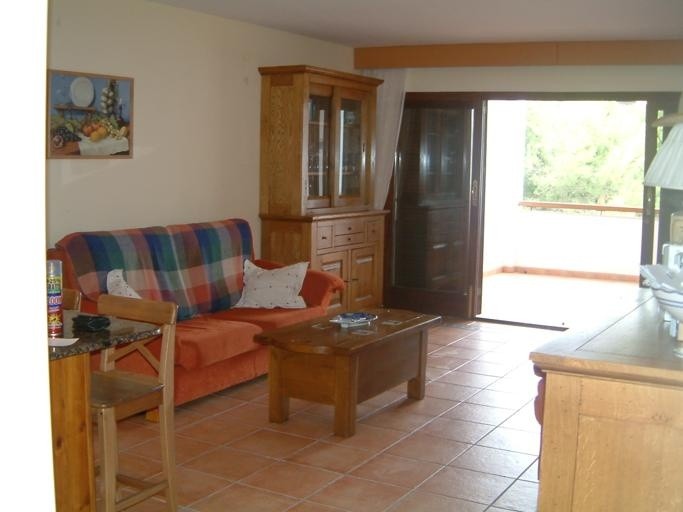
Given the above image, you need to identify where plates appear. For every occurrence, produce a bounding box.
[328,311,377,328]
[67,77,95,107]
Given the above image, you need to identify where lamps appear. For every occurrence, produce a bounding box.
[641,123,682,195]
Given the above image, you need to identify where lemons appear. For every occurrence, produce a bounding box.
[91,132,99,140]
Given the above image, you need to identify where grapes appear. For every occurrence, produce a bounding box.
[55,128,81,141]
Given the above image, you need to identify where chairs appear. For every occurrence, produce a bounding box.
[88,292,181,512]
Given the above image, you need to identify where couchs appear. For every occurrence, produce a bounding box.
[48,217,347,411]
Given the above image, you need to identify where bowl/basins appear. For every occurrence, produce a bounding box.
[641,279,682,325]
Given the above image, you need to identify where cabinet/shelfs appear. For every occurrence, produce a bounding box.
[260,210,391,307]
[529,282,681,512]
[259,64,384,215]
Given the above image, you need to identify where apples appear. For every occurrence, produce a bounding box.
[83,126,93,137]
[92,123,98,130]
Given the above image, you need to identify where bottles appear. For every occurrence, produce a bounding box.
[46,259,63,339]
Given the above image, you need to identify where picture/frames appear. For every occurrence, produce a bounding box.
[46,67,135,160]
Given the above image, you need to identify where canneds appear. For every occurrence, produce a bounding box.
[47,296,63,337]
[46,259,63,297]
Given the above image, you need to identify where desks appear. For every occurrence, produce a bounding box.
[253,305,442,439]
[48,305,162,512]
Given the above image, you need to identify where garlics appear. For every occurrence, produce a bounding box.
[101,87,114,113]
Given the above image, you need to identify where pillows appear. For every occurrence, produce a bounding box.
[106,268,142,299]
[230,259,310,309]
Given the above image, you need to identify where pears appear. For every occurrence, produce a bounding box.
[97,127,106,137]
